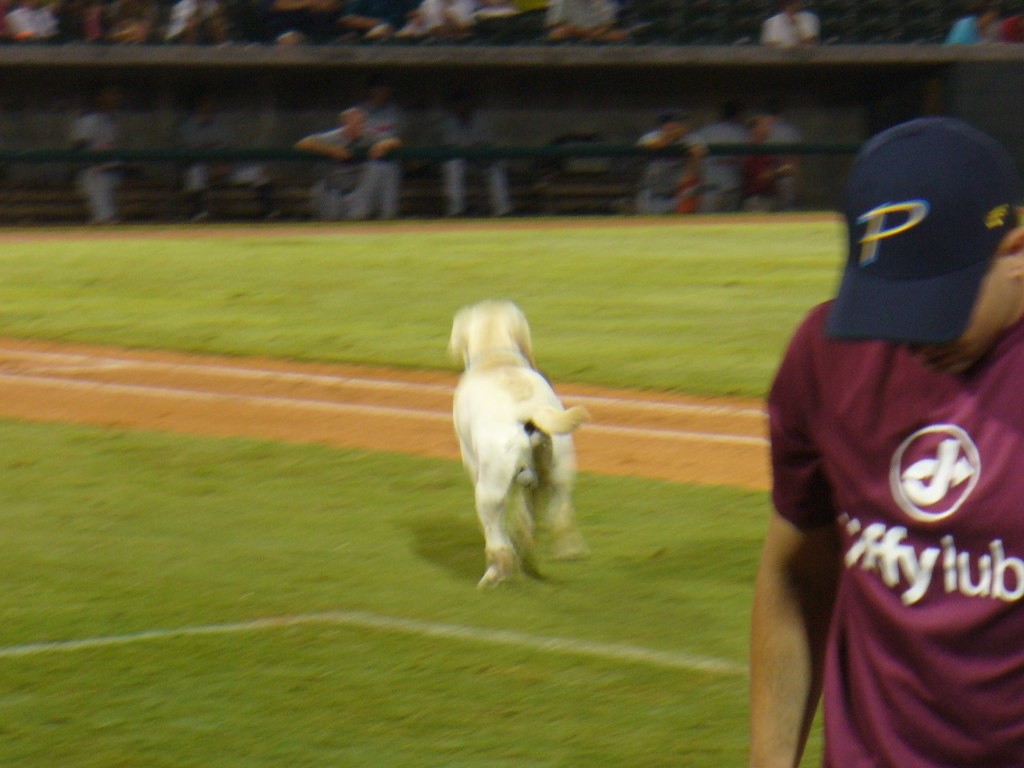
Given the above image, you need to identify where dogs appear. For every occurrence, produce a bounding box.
[446,300,592,593]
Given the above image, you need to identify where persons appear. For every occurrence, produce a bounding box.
[65,91,121,225]
[175,98,227,221]
[941,7,1024,45]
[439,103,515,219]
[218,125,282,222]
[761,0,821,46]
[0,0,514,45]
[748,116,1024,768]
[292,83,404,221]
[632,99,802,215]
[544,0,627,45]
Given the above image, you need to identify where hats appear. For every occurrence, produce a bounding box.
[827,118,1020,342]
